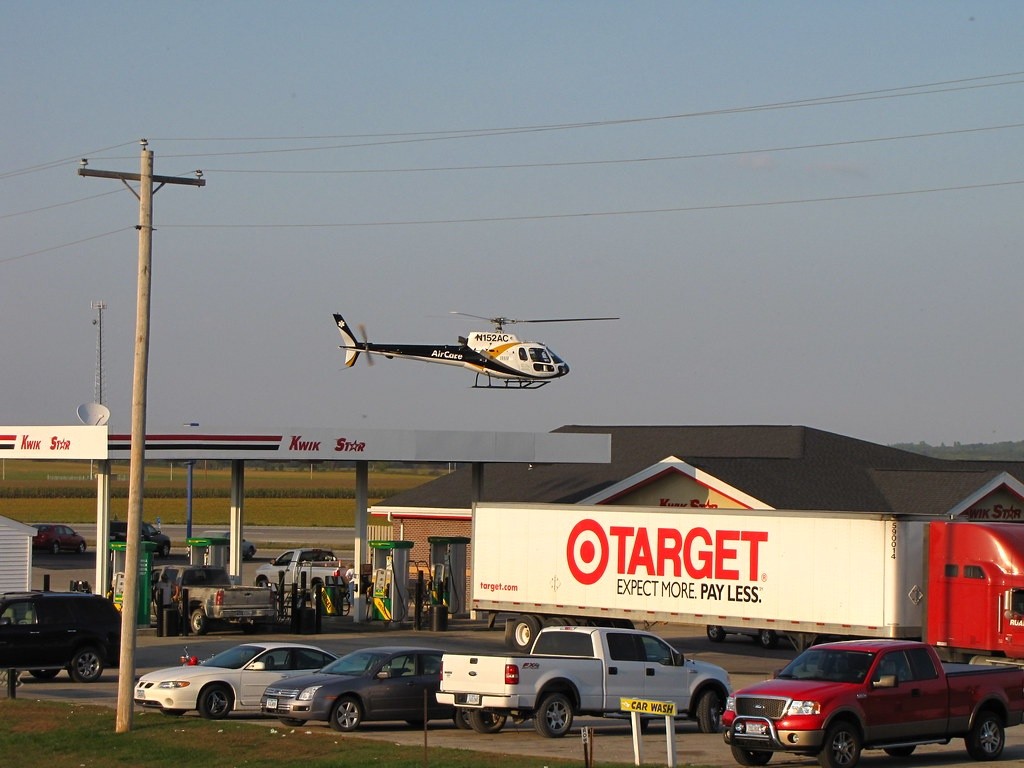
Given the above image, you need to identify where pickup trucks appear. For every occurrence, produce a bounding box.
[435,624,732,739]
[151,562,276,637]
[253,548,355,606]
[722,639,1024,768]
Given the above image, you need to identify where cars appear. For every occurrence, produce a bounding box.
[260,644,473,733]
[186,529,257,562]
[133,642,351,721]
[706,624,819,649]
[29,524,88,557]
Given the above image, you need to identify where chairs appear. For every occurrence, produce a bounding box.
[402,659,414,677]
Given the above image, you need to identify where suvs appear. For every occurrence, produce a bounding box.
[0,590,121,684]
[108,519,171,559]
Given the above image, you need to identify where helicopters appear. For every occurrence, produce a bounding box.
[333,311,620,389]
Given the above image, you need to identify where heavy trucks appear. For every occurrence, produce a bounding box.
[470,499,1024,660]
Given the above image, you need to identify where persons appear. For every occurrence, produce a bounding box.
[152,573,180,617]
[366,582,373,618]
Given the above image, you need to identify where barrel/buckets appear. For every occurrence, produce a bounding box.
[157,607,179,637]
[429,605,447,631]
[296,606,315,635]
